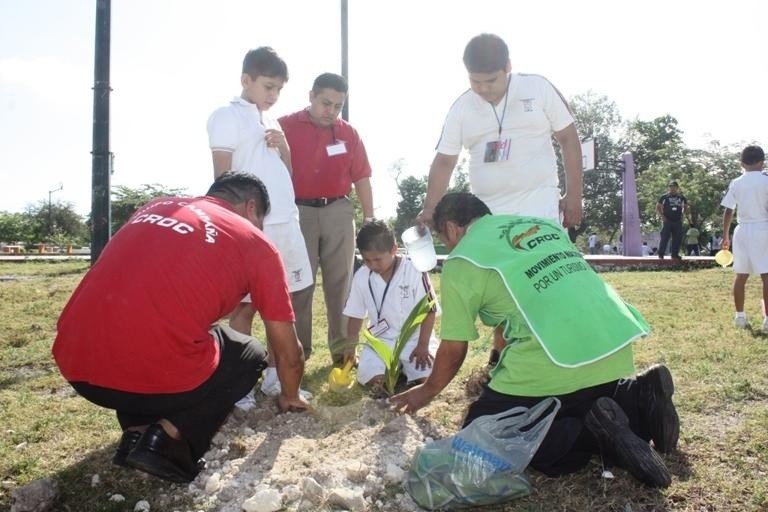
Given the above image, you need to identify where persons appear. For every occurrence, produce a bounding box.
[52,169,310,484]
[277,72,374,368]
[343,219,442,387]
[388,192,680,489]
[415,33,584,377]
[206,45,313,414]
[587,146,768,330]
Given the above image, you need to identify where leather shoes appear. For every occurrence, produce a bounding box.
[124,424,206,483]
[635,365,680,454]
[112,430,141,468]
[590,396,673,488]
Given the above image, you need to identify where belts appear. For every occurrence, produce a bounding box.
[296,196,339,208]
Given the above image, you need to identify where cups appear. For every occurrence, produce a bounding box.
[402,226,437,272]
[714,249,733,267]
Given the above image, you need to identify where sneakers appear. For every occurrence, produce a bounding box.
[260,376,313,400]
[760,319,768,334]
[735,316,748,328]
[467,363,497,393]
[234,388,257,411]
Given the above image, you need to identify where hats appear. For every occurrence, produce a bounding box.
[669,182,677,186]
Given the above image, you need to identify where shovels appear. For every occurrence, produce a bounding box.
[328,359,356,394]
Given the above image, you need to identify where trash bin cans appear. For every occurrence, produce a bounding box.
[67,245,72,255]
[39,244,45,254]
